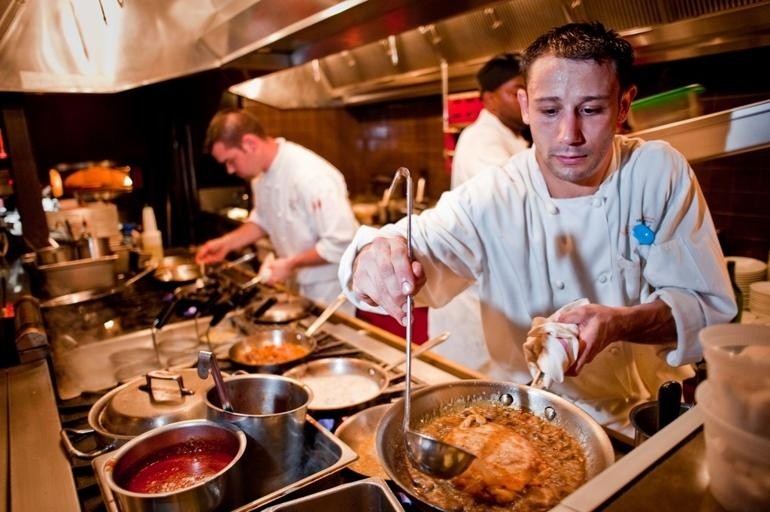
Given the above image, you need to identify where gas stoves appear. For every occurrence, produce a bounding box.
[57,327,417,512]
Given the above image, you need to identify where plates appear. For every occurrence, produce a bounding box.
[723,254,770,322]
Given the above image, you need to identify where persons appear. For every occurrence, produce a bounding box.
[422,51,527,375]
[337,20,739,440]
[195,108,360,320]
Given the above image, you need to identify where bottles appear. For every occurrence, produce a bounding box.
[140,206,164,261]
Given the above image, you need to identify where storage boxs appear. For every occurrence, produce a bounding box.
[626,85,705,129]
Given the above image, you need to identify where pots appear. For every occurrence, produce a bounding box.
[279,332,452,420]
[375,367,617,512]
[153,251,258,290]
[75,236,113,259]
[227,290,347,373]
[39,263,157,315]
[36,244,79,266]
[175,276,261,312]
[104,417,250,512]
[334,401,400,481]
[202,372,314,475]
[626,399,693,452]
[60,366,252,461]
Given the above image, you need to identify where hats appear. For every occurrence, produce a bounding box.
[477,53,528,100]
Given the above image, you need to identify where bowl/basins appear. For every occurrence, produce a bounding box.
[694,378,770,512]
[695,323,770,436]
